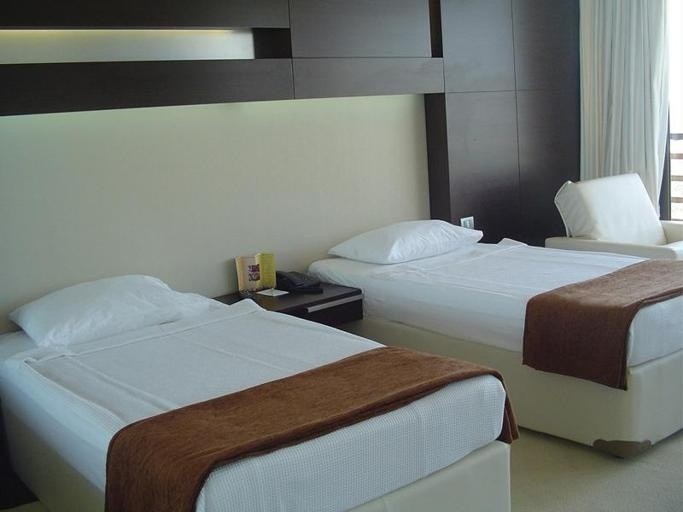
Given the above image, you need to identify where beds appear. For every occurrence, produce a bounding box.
[0,294,512,510]
[307,219,681,458]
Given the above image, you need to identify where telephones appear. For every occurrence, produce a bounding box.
[276,271,323,294]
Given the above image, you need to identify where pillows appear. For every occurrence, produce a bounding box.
[6,273,180,350]
[327,218,486,265]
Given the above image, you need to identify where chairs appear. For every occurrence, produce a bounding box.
[541,172,681,261]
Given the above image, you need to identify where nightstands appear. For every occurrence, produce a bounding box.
[210,272,361,326]
